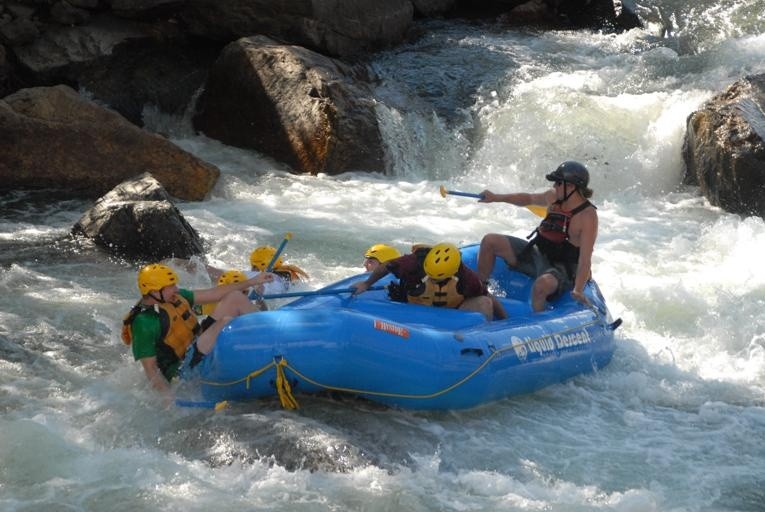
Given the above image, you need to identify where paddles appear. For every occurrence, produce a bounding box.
[440,185,547,218]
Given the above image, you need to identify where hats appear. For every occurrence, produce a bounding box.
[546,171,563,182]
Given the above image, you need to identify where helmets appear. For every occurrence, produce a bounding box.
[364,244,401,265]
[217,268,249,291]
[250,246,283,272]
[137,263,177,296]
[556,161,589,188]
[423,242,461,280]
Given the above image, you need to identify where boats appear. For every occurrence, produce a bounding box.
[199,244,623,411]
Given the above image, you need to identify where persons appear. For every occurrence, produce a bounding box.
[190,270,269,313]
[472,160,599,314]
[249,246,306,292]
[363,243,402,272]
[349,241,509,322]
[120,262,273,410]
